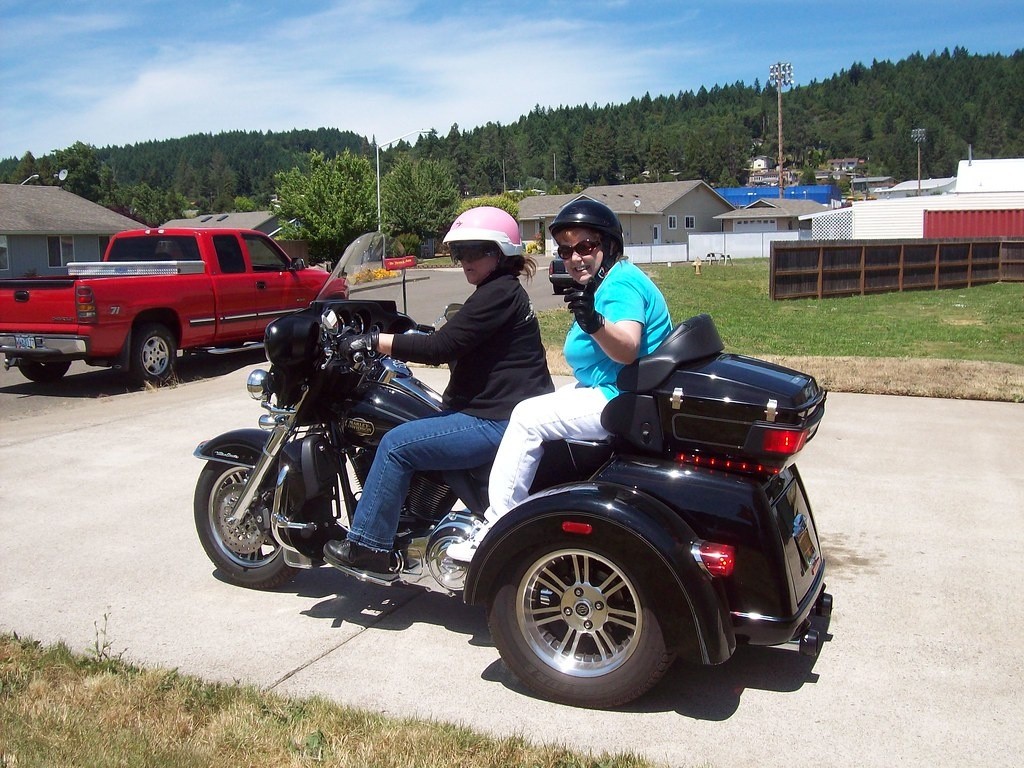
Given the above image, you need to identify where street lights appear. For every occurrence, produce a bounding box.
[910,128,927,198]
[769,62,795,200]
[376,130,432,232]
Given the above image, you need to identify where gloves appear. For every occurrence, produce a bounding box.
[340,330,379,365]
[563,277,605,335]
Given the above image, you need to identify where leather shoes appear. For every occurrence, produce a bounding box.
[323,536,400,581]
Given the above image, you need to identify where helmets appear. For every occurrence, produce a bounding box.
[442,206,524,257]
[549,200,624,256]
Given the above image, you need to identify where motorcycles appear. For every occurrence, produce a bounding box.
[193,232,835,712]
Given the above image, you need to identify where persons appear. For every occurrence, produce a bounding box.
[447,200,674,561]
[322,204,555,569]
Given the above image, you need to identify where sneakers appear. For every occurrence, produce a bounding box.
[446,522,492,562]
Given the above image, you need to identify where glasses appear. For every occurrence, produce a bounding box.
[447,244,500,262]
[557,239,601,260]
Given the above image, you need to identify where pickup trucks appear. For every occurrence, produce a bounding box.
[0,228,351,387]
